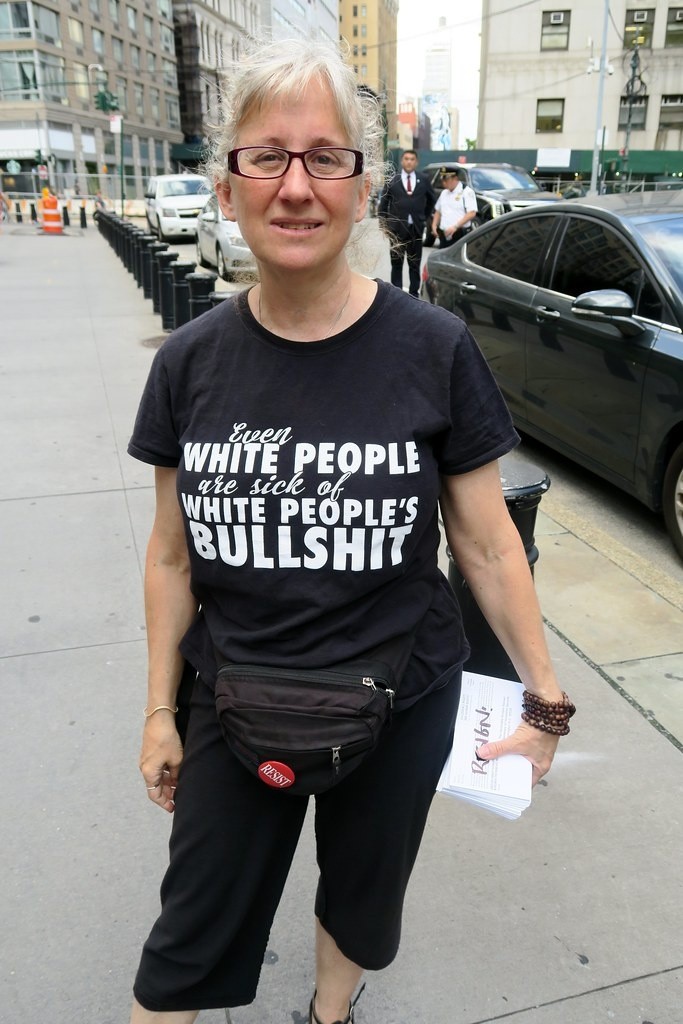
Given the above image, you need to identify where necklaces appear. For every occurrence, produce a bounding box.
[259,280,350,339]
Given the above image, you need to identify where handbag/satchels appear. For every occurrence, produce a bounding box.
[210,658,391,797]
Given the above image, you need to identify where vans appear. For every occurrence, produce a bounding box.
[143,173,215,243]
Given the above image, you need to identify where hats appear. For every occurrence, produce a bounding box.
[438,165,460,179]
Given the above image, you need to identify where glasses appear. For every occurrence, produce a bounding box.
[226,144,365,180]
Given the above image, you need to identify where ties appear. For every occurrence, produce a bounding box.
[406,174,412,192]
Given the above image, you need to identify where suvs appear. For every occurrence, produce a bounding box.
[422,162,565,237]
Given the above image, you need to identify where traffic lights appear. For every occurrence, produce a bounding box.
[34,150,42,164]
[93,91,119,115]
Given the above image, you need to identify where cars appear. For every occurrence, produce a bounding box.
[419,190,683,562]
[196,193,260,282]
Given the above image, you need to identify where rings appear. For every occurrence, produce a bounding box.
[145,782,159,789]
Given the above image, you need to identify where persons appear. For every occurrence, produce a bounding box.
[377,151,435,298]
[432,167,478,249]
[128,39,577,1024]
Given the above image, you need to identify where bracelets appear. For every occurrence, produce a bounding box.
[521,690,576,736]
[143,706,178,716]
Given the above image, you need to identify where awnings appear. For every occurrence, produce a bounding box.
[389,149,683,176]
[170,143,217,163]
